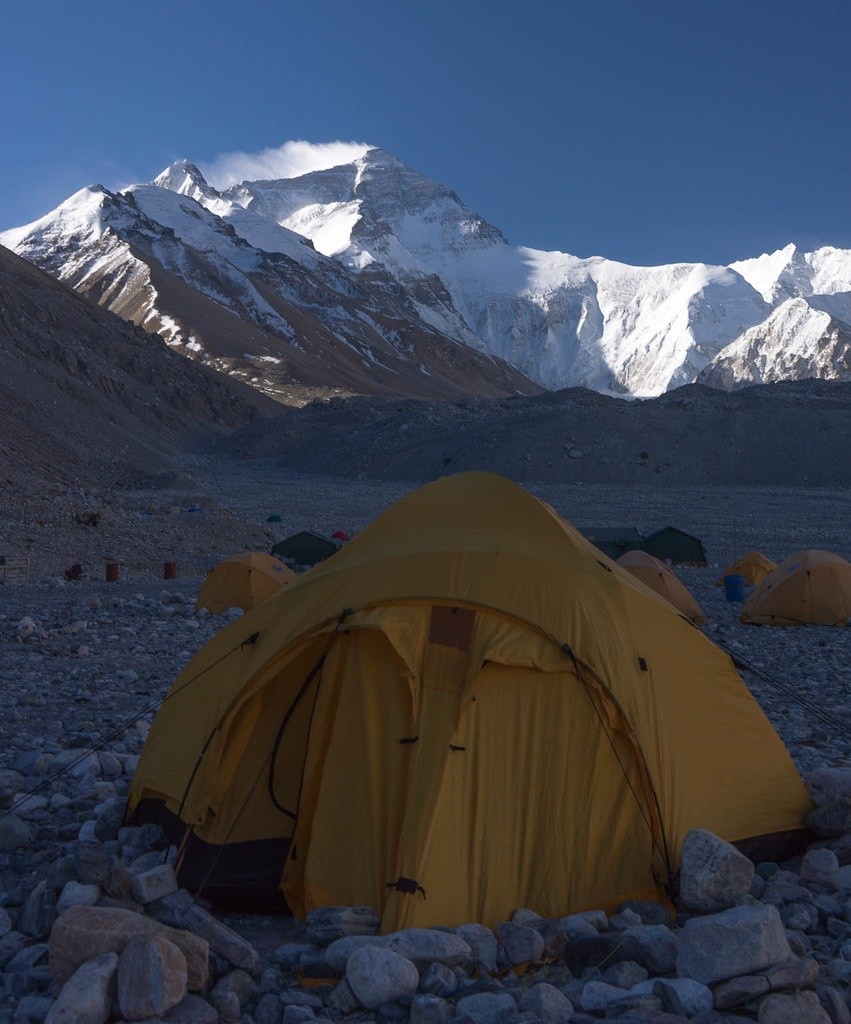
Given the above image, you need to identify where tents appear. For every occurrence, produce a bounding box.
[718,550,779,588]
[196,551,297,614]
[576,526,645,563]
[614,549,706,627]
[741,546,851,630]
[270,528,343,572]
[124,471,813,941]
[645,525,707,565]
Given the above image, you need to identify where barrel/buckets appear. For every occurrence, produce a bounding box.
[724,574,745,602]
[164,562,176,578]
[106,564,118,582]
